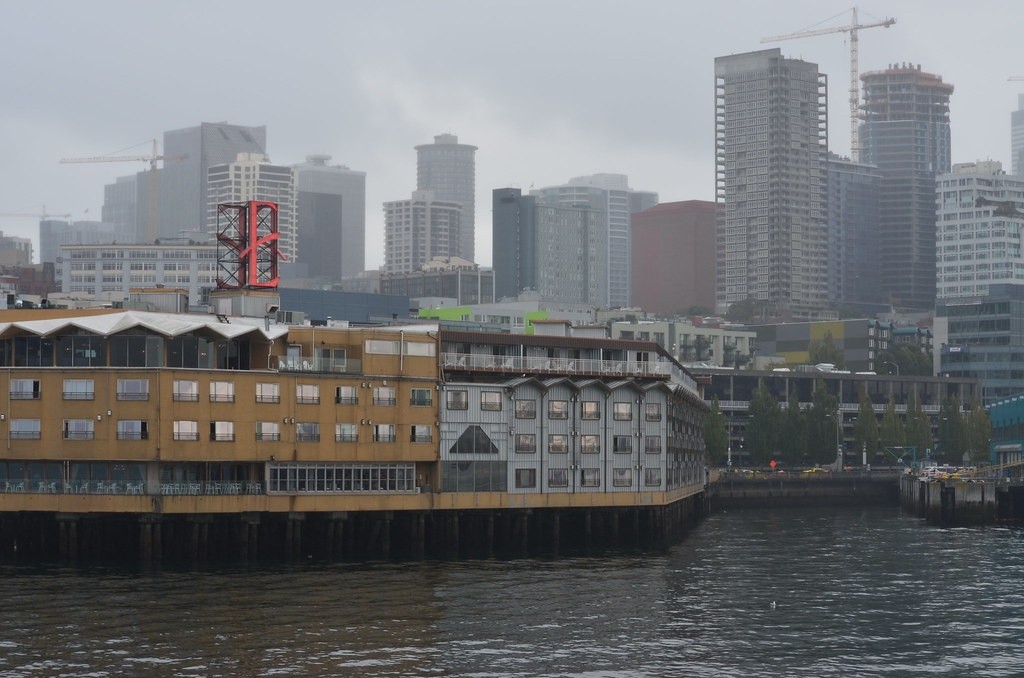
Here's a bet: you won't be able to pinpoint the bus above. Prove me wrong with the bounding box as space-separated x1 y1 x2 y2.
923 467 977 476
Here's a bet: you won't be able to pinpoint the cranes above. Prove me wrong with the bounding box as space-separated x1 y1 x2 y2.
58 138 190 245
759 6 897 163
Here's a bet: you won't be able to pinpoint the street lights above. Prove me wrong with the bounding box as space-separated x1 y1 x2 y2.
826 414 839 456
913 418 919 446
933 418 947 461
884 361 899 375
717 412 731 472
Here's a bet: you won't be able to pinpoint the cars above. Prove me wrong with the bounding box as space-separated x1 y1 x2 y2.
800 468 831 475
843 463 862 471
